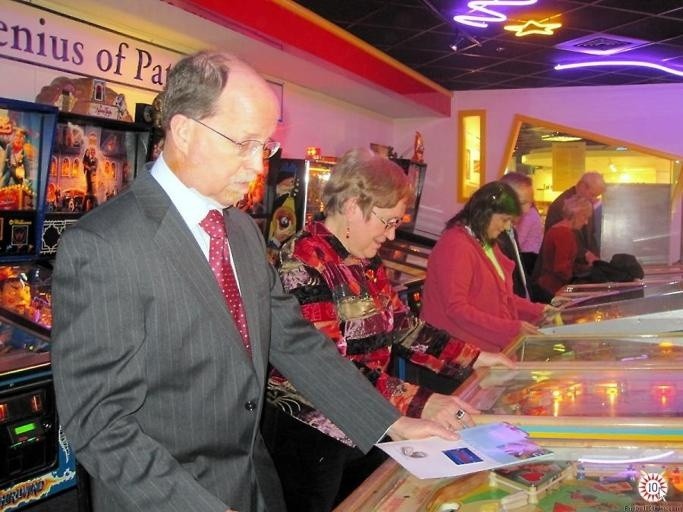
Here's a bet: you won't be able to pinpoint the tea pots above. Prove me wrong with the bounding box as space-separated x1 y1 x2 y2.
457 108 486 203
500 114 683 216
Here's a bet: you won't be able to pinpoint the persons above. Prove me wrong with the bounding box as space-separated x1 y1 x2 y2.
0 130 30 187
513 203 544 297
90 149 97 195
51 50 461 512
544 172 606 267
259 146 518 512
531 193 593 305
82 148 92 195
421 181 561 392
496 173 555 304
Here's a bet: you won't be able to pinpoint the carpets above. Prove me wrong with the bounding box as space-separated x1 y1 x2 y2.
199 209 251 361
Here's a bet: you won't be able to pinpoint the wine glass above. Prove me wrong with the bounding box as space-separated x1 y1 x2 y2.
456 408 466 420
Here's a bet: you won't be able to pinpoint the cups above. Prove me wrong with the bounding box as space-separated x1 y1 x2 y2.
182 112 280 159
487 182 506 201
369 210 403 230
